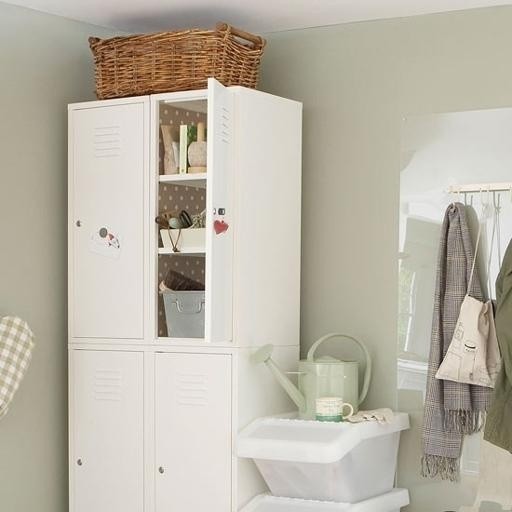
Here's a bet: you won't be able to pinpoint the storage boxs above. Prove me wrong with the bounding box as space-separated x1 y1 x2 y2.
235 412 410 512
158 290 205 338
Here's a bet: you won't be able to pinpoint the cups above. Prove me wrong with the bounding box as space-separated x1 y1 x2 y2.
315 396 354 422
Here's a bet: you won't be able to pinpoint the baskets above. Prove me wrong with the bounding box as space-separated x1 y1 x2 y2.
87 20 267 100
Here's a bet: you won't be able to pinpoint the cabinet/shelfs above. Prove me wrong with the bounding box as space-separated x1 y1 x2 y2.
67 78 305 512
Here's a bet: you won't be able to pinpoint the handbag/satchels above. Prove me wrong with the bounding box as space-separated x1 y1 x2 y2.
433 293 504 391
